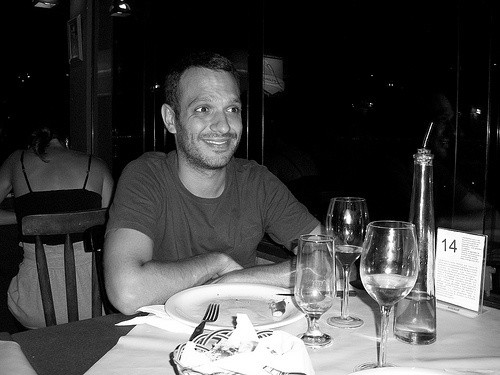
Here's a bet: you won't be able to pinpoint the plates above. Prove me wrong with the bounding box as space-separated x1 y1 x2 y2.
164 283 306 332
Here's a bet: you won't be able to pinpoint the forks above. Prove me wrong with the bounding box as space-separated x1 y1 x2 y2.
189 303 220 341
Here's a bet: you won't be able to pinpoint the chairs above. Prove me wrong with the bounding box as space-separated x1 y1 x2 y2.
22 208 109 326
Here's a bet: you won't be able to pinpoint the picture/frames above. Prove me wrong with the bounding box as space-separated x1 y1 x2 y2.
66 12 83 65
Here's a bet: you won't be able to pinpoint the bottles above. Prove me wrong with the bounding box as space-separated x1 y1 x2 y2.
393 149 438 346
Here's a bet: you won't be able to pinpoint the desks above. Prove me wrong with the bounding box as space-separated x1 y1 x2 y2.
0 281 500 375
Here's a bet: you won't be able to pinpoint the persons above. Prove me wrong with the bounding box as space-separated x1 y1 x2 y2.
0 112 113 329
271 130 321 214
427 95 500 230
104 54 354 316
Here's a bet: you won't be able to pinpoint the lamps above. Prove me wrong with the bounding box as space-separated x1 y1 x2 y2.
108 0 134 17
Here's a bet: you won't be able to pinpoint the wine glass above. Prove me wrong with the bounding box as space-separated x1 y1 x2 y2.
324 197 369 328
294 233 337 347
353 220 419 372
